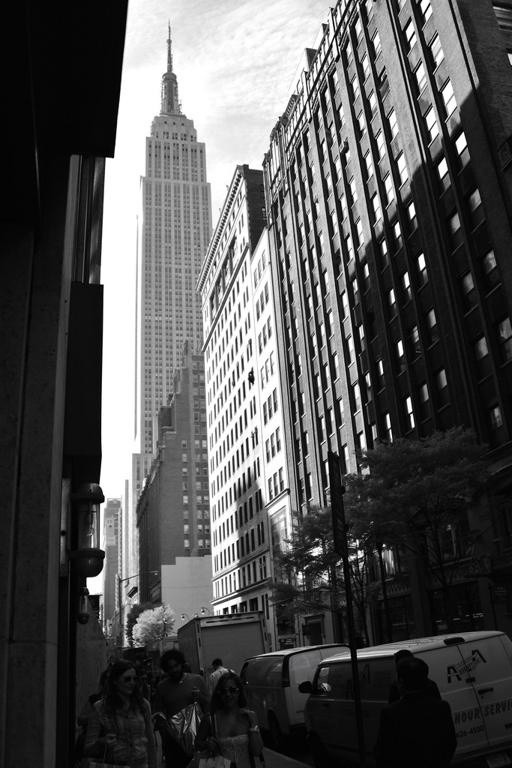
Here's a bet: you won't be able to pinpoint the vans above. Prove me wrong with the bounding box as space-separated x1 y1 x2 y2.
238 644 351 750
297 631 512 764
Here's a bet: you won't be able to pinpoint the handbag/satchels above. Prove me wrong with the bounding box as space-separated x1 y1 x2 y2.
198 752 232 768
164 702 206 755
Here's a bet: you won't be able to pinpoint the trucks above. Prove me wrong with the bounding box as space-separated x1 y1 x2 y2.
142 636 179 680
177 610 271 687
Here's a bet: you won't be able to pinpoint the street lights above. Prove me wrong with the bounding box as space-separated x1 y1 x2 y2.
117 571 159 646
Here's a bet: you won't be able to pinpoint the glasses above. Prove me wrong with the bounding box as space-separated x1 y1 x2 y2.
218 688 240 695
123 675 138 683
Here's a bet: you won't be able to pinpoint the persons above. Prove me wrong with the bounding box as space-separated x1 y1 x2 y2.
372 650 458 767
72 644 267 767
